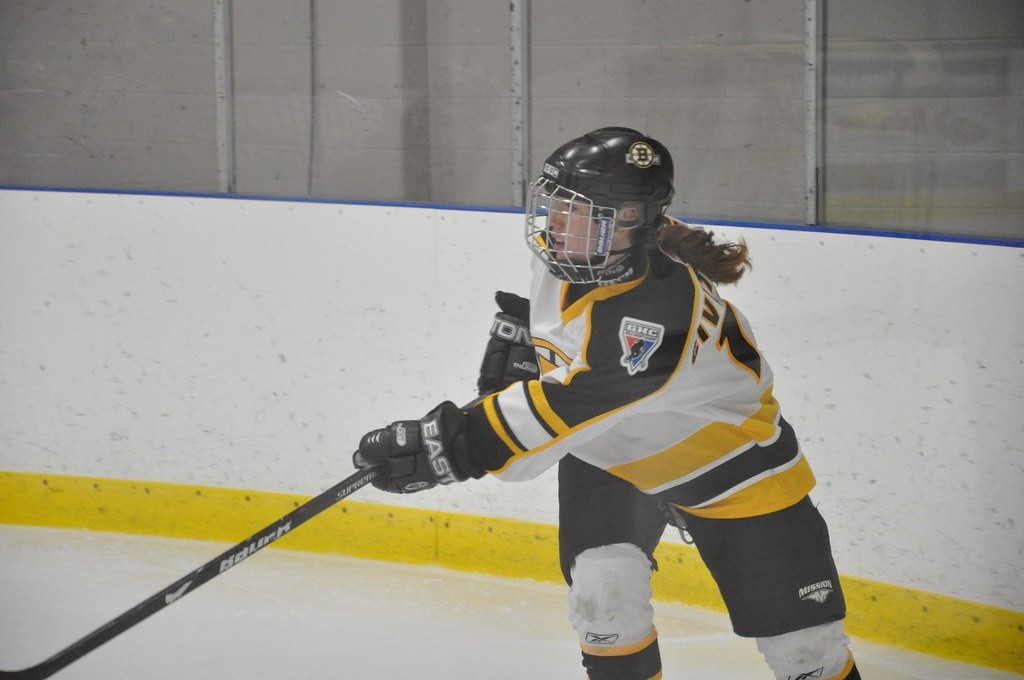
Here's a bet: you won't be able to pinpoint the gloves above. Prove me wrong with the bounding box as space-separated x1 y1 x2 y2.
477 291 540 399
353 401 487 495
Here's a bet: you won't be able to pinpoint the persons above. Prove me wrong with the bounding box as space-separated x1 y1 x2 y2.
352 126 861 680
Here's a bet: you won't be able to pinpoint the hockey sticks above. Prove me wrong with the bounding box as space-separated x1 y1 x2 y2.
0 386 499 680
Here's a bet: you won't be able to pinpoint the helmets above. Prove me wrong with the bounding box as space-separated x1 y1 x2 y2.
526 125 674 284
